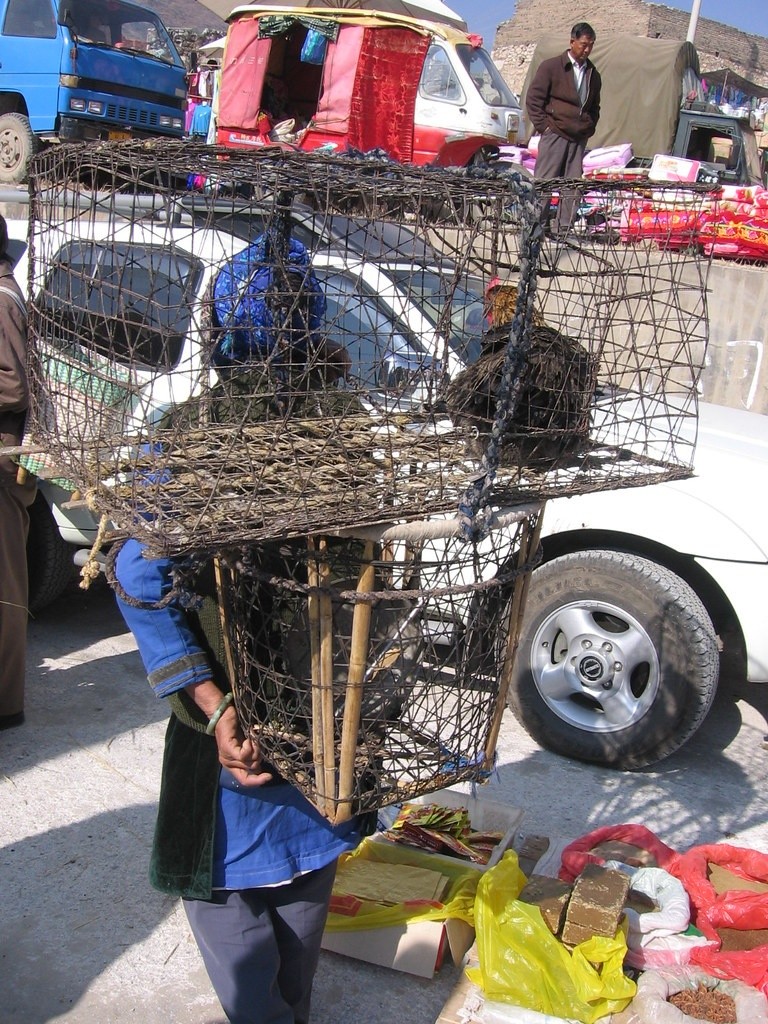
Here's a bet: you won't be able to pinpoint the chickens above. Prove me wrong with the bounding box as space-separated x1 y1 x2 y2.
445 278 611 451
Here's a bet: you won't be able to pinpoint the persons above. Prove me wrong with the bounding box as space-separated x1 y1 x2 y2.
525 22 602 243
82 10 107 45
105 233 405 1023
0 214 47 730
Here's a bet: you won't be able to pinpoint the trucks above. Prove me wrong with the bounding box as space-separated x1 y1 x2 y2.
0 1 198 185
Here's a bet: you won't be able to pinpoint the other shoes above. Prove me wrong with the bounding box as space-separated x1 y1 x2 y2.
0 712 25 731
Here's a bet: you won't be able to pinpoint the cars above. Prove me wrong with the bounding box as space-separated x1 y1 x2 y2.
0 195 767 773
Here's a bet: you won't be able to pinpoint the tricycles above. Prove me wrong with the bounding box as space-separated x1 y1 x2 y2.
214 6 540 236
518 30 764 191
